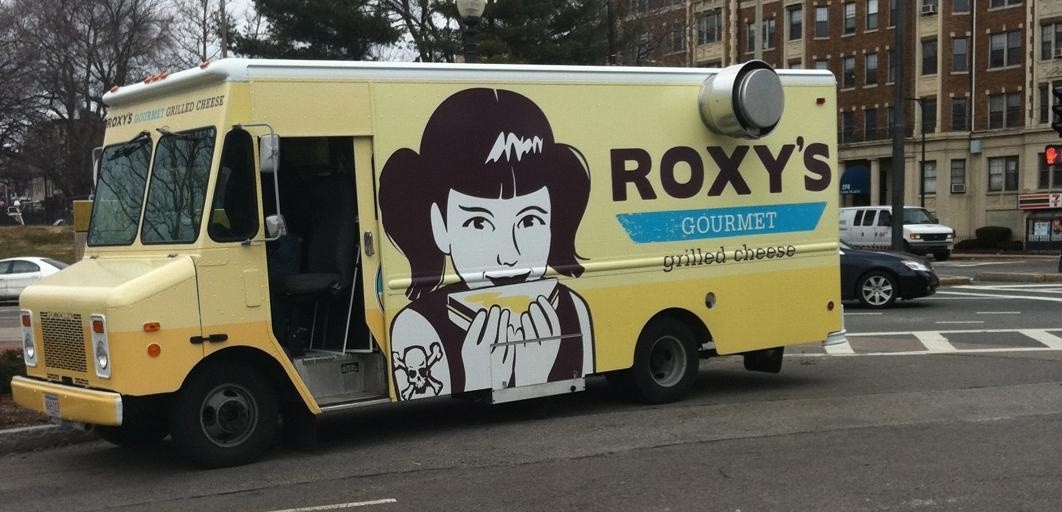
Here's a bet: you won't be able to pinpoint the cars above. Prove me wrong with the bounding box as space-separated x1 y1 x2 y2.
0 256 69 303
840 239 940 309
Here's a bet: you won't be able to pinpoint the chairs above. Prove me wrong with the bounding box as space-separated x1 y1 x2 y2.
269 175 359 349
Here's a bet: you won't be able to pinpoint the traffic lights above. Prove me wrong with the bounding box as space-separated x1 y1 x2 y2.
1045 145 1058 165
1051 87 1062 137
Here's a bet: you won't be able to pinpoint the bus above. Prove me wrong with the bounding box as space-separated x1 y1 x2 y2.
11 57 846 468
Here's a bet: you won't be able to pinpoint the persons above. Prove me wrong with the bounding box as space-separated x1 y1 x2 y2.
372 86 598 406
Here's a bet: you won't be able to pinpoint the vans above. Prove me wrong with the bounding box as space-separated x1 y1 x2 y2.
839 206 956 261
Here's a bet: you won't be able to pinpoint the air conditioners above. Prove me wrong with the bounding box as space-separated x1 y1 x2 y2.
920 4 937 14
951 184 965 193
